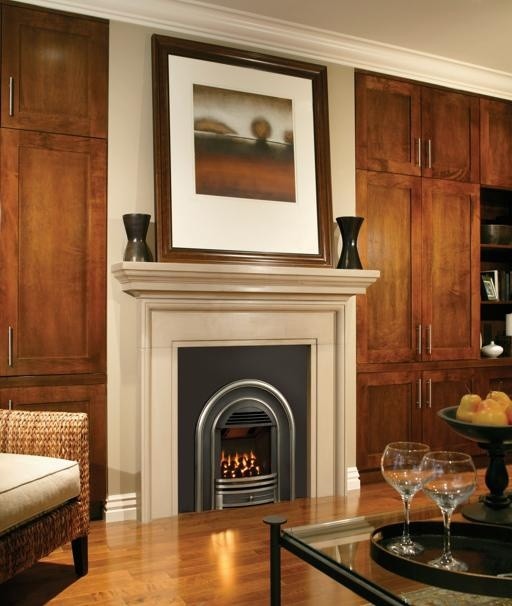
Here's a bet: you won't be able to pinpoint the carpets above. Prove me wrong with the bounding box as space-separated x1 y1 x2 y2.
364 586 512 606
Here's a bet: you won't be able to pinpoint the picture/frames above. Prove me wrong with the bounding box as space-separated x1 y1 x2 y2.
152 34 334 269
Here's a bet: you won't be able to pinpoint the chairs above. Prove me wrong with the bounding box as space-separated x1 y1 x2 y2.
0 410 90 585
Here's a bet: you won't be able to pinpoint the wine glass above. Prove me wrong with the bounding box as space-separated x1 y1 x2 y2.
436 406 512 525
380 441 430 560
420 450 479 573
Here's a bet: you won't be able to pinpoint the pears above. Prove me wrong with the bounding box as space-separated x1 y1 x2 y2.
457 383 512 425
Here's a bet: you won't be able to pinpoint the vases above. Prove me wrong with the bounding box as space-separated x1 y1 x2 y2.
123 214 153 262
336 217 364 269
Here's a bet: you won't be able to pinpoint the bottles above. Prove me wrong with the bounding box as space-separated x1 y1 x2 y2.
482 340 504 360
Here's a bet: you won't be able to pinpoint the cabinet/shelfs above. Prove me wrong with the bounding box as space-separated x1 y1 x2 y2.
1 0 109 520
355 71 512 484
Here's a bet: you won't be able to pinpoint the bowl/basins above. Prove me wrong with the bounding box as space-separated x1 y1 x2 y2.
480 224 512 245
